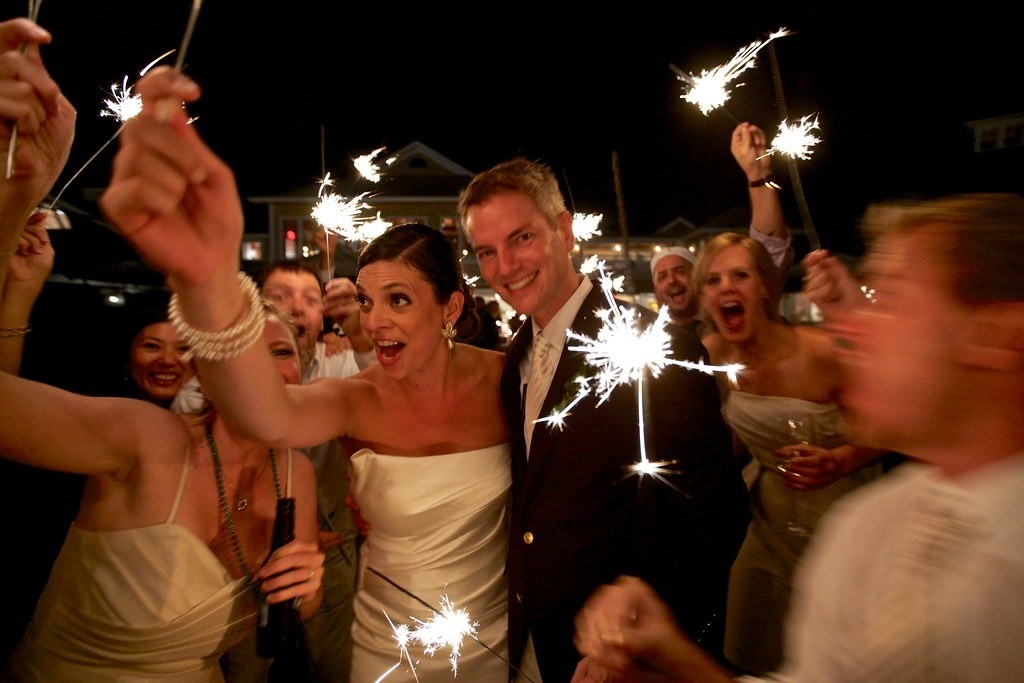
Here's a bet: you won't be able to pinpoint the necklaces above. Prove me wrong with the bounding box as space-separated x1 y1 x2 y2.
169 272 267 361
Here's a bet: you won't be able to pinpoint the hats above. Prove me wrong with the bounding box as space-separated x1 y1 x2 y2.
650 246 696 275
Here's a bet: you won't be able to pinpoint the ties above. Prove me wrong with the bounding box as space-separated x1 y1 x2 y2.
524 331 556 462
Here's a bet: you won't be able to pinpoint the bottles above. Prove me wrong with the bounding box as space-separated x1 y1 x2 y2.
256 494 298 659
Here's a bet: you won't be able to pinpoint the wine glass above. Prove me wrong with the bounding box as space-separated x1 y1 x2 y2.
774 411 816 537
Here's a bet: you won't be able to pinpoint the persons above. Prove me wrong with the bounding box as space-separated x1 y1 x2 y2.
651 120 910 682
105 65 541 682
459 159 754 683
0 209 378 683
580 189 1024 680
3 9 79 289
